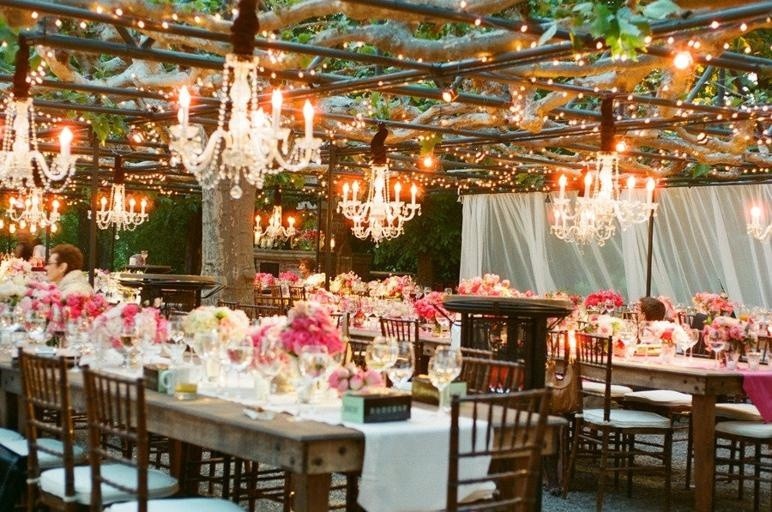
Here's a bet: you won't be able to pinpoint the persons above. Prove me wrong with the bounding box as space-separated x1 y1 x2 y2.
637 296 665 323
298 256 316 280
0 238 93 300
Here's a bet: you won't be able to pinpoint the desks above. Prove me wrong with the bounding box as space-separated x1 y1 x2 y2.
0 313 569 511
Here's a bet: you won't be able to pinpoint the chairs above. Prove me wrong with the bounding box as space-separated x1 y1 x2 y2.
15 345 183 510
80 363 248 510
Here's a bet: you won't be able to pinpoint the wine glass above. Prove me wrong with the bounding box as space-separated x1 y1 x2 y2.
574 300 725 369
0 306 462 421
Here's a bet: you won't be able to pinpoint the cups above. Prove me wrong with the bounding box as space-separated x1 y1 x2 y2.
746 352 761 370
724 353 741 369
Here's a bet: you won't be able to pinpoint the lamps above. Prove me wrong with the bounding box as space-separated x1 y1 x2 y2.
333 164 425 251
443 75 465 103
0 99 81 195
164 44 325 201
0 182 62 238
85 182 149 240
253 205 298 250
547 149 660 248
745 198 772 244
